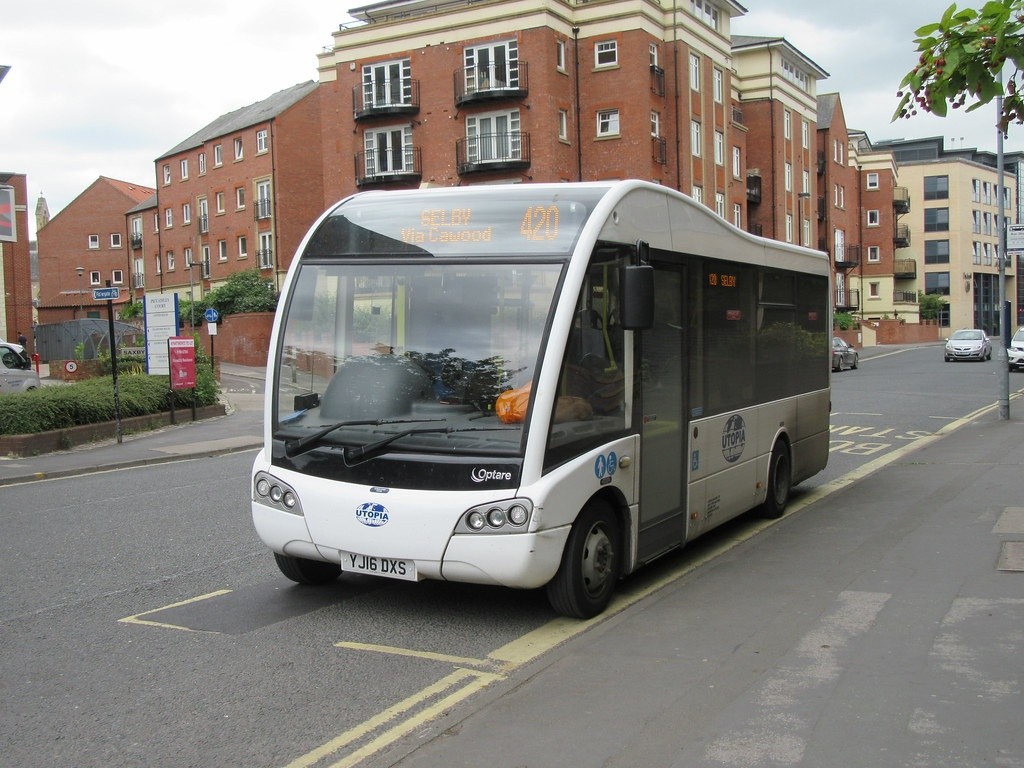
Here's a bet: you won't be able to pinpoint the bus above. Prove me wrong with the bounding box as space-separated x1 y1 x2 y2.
251 179 834 620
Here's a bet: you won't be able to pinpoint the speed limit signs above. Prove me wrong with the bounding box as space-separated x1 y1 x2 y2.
66 361 78 373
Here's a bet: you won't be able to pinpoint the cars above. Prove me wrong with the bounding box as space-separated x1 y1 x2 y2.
944 329 992 362
833 337 858 372
1007 327 1024 372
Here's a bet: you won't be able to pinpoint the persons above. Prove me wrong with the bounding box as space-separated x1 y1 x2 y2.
17 331 27 349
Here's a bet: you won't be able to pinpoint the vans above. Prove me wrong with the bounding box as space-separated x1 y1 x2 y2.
0 343 40 396
